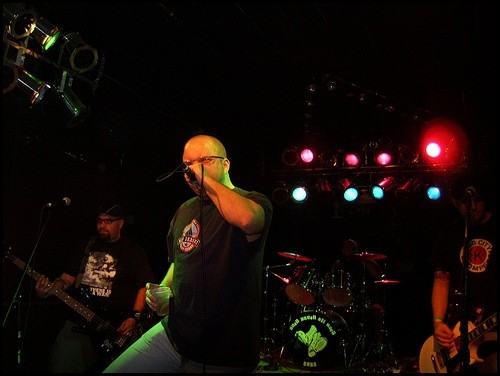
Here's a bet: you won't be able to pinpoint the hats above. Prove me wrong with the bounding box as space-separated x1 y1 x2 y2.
95 201 124 216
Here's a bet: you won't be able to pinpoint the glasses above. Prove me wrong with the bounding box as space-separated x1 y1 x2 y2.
183 155 225 166
96 217 123 225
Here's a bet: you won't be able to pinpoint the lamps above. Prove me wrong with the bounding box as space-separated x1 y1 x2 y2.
0 0 99 130
289 139 442 204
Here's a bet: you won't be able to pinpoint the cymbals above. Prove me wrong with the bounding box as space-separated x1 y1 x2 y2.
374 279 400 284
353 253 388 261
276 251 314 262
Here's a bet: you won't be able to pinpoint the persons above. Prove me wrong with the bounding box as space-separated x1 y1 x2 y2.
432 180 497 373
320 238 384 345
35 204 155 374
103 135 272 374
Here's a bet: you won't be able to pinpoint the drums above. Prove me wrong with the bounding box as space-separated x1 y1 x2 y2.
282 307 351 371
322 267 357 306
342 307 386 353
284 265 327 305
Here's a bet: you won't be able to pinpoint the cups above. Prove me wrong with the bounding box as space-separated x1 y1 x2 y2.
150 287 172 316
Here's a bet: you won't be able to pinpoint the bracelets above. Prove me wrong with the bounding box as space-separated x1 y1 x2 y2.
130 310 142 321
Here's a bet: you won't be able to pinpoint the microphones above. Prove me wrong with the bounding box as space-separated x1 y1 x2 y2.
181 163 204 193
48 197 71 207
464 186 477 200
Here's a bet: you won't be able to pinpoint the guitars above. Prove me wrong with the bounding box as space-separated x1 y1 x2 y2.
418 312 498 374
0 244 147 365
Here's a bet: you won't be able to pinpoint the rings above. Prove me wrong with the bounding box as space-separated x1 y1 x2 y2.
127 332 131 336
